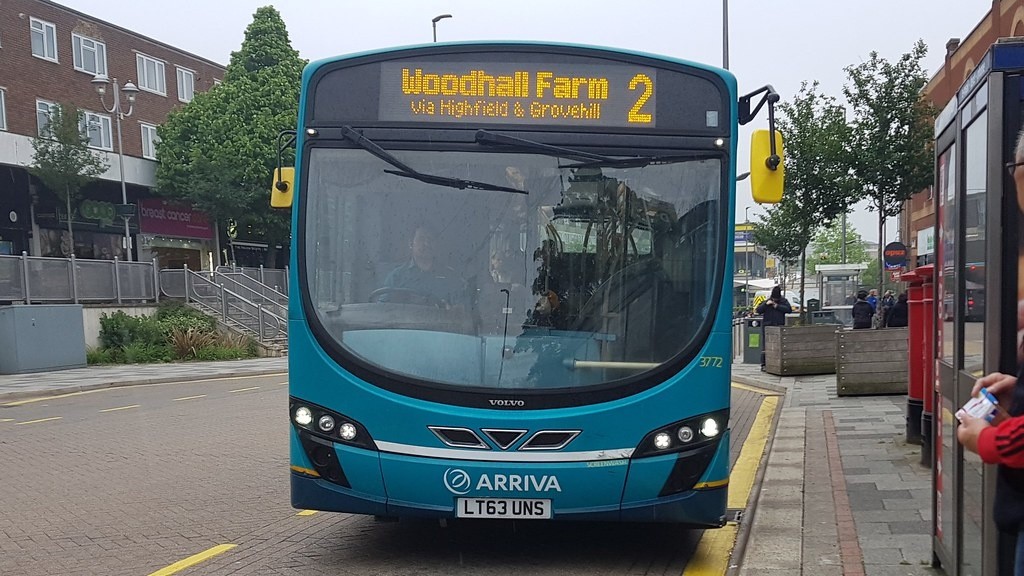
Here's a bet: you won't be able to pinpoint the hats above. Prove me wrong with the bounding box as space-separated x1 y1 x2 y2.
858 290 869 299
772 287 781 299
899 293 908 301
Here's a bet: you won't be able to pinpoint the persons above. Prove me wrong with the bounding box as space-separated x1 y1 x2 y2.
380 225 470 304
836 290 908 329
490 249 518 283
757 286 792 372
956 129 1024 576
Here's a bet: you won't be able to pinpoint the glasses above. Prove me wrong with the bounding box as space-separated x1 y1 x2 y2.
1006 161 1024 176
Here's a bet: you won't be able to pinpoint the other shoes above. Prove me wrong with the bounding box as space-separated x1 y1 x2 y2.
761 366 766 371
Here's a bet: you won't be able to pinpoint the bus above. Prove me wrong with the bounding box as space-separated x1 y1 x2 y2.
271 35 784 530
944 264 986 323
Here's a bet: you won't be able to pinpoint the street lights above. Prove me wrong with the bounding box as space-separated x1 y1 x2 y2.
91 71 140 261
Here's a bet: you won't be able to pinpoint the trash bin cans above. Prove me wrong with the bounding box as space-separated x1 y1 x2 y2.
813 311 838 326
744 317 764 363
807 298 821 322
